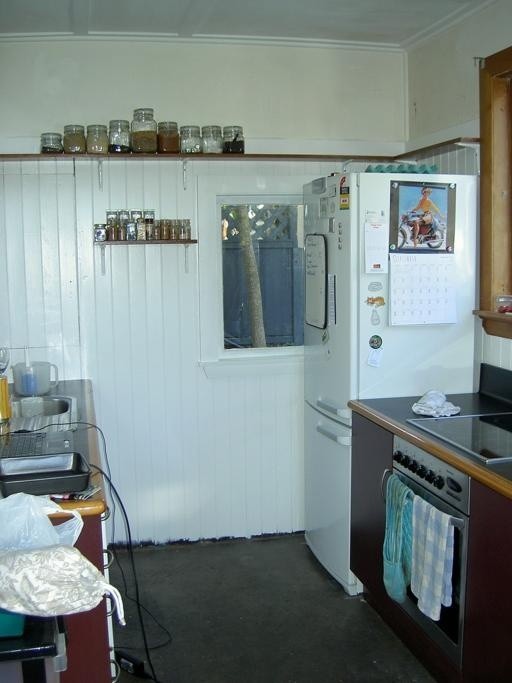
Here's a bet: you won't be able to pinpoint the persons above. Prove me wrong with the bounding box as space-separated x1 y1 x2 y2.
408 187 444 247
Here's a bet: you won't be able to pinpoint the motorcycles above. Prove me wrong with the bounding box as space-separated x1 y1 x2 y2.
398 210 444 248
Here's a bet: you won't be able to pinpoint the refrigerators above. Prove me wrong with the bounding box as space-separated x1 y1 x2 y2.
301 171 478 596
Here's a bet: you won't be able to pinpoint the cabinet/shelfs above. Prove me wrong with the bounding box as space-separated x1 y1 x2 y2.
0 152 399 244
0 377 122 683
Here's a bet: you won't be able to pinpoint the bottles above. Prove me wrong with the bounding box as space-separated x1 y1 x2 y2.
93 209 192 242
38 105 245 154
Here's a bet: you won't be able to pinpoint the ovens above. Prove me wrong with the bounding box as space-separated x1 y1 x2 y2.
385 433 472 673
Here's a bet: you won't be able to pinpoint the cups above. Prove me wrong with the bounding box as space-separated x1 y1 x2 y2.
10 360 60 397
20 396 44 416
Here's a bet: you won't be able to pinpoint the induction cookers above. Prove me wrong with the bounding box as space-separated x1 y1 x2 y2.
404 410 512 465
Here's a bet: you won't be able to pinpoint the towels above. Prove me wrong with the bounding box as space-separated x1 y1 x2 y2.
410 388 461 419
381 474 456 623
